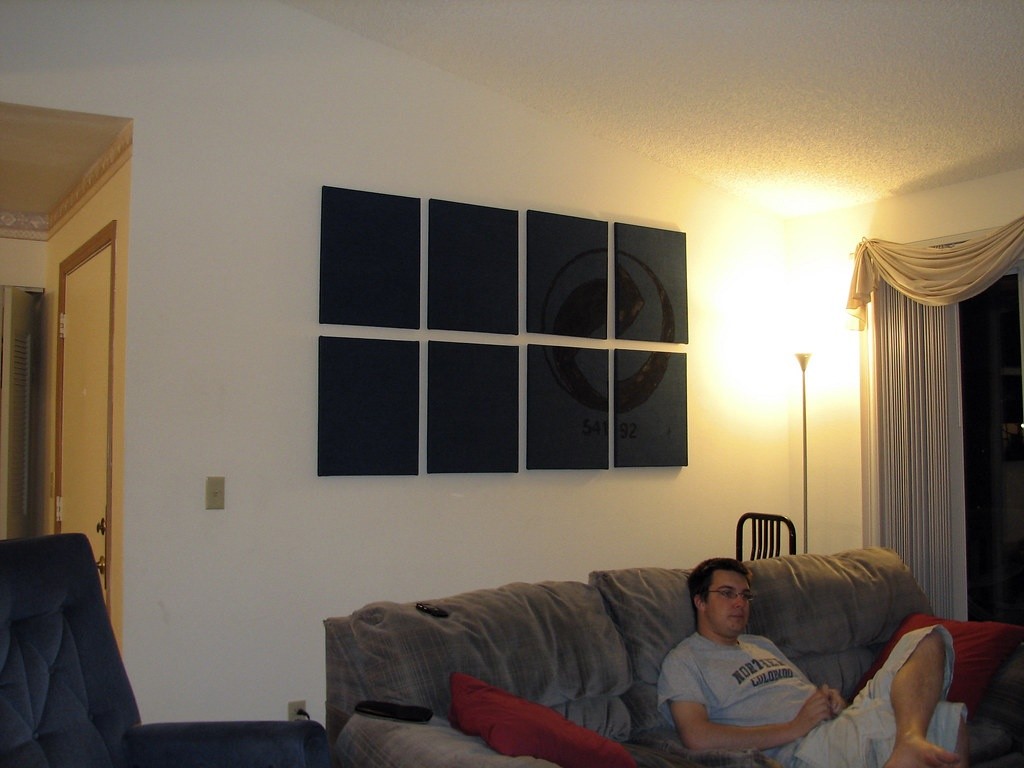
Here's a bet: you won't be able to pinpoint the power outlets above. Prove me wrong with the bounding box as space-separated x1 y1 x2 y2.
288 700 306 721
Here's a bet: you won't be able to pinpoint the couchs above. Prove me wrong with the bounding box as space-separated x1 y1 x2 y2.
322 545 1024 767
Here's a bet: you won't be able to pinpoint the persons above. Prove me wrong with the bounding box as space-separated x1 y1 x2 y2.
658 558 968 768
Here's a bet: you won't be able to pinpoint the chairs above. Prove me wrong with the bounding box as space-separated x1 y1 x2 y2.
0 533 331 768
734 513 797 560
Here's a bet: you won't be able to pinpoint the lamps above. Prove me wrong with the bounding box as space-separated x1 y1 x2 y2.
795 352 814 557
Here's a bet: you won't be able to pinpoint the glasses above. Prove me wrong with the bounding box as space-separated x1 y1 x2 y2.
709 589 754 601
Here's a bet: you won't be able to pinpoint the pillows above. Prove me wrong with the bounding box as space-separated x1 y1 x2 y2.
852 613 1024 712
444 670 637 768
